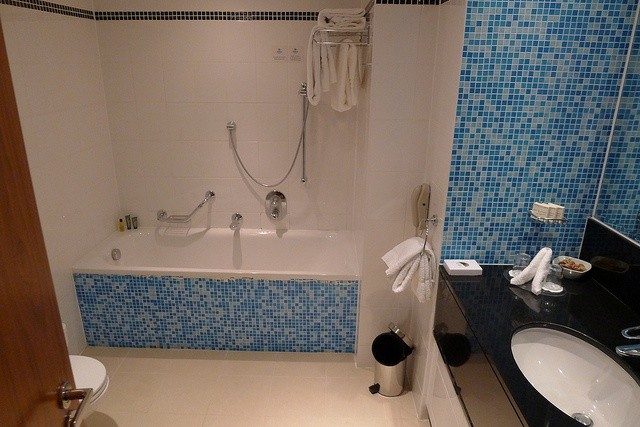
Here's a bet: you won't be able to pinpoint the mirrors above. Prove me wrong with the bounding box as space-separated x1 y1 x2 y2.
592 0 640 247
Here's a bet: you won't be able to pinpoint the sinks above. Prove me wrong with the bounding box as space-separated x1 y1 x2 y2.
511 322 639 427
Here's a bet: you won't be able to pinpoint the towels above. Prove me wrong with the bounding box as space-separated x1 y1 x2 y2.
316 8 367 30
306 26 335 106
508 246 552 295
391 253 431 303
380 236 437 287
332 38 358 112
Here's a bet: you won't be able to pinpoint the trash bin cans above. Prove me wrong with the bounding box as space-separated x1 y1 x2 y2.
369 320 415 396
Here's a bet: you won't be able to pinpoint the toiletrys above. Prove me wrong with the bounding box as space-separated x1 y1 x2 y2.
118 218 125 232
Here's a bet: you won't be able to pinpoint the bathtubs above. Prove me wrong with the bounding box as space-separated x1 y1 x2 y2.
73 226 359 354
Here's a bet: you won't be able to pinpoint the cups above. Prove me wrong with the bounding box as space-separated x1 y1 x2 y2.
514 253 530 278
543 265 562 292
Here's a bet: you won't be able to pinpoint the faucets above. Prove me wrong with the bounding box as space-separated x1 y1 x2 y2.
616 344 640 356
229 213 244 230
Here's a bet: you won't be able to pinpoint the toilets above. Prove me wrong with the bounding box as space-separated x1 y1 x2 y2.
62 323 110 419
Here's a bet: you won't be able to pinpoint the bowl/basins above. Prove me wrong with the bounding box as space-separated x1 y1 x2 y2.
553 255 591 277
